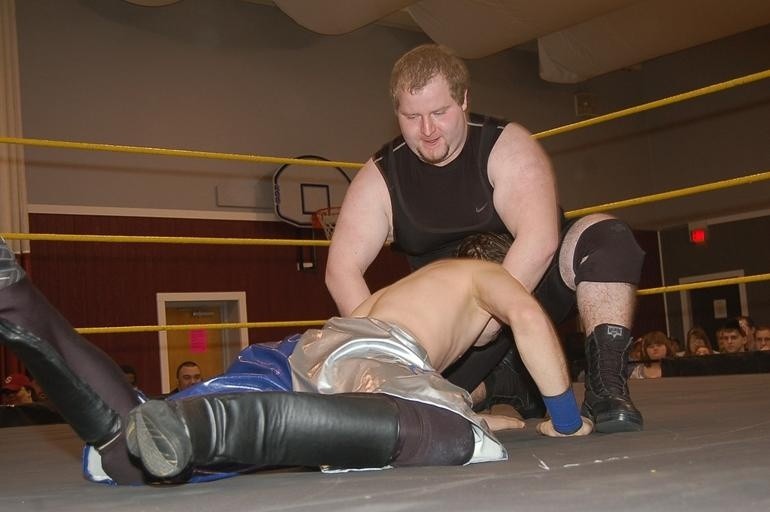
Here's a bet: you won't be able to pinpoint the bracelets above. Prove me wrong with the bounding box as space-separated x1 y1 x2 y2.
538 382 583 435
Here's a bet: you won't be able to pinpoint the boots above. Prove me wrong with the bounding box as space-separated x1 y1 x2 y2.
126 391 400 479
0 237 148 457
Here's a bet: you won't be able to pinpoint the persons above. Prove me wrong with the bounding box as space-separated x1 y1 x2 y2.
0 371 41 408
318 43 644 434
120 362 141 391
0 231 595 495
171 360 202 392
629 310 769 378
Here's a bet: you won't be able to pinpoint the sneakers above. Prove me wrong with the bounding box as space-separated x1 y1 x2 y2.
581 321 647 432
479 345 553 421
